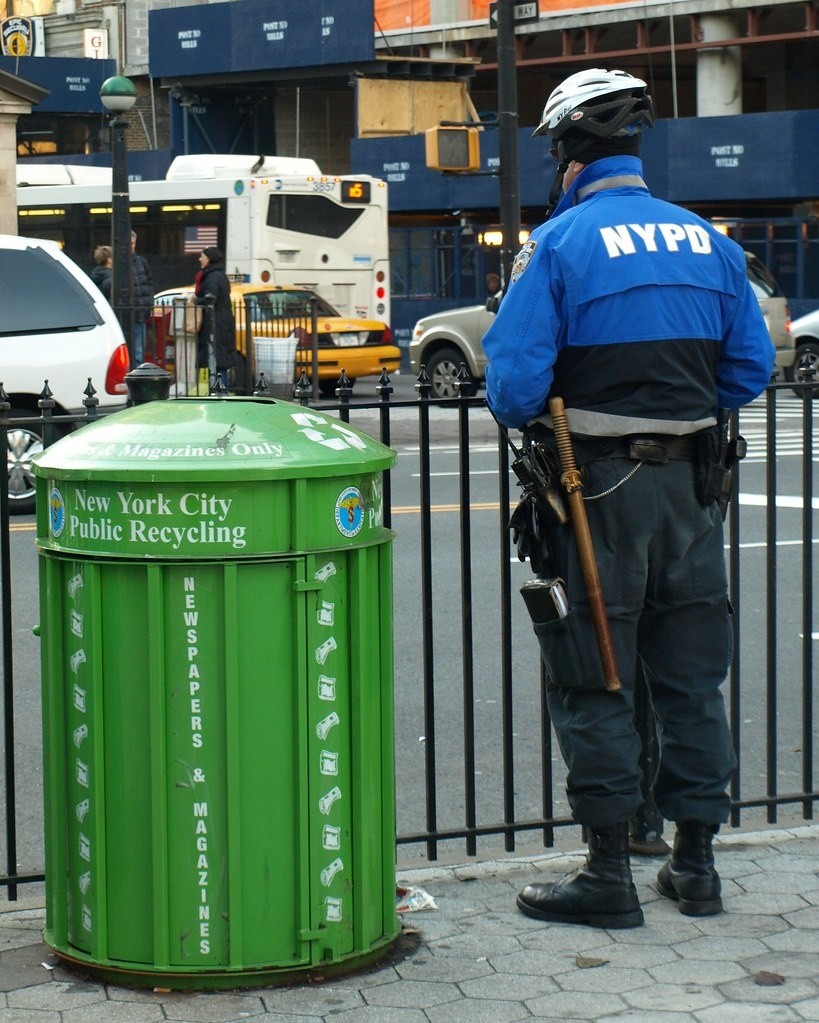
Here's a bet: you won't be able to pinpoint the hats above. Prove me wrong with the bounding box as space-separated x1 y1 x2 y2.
203 246 223 264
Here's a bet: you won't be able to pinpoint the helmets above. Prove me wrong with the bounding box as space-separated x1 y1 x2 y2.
531 68 655 139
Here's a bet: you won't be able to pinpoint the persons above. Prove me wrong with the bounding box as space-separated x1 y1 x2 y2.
88 230 153 370
188 247 237 397
481 68 777 926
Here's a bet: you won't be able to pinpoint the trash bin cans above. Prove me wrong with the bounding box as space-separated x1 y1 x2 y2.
30 396 401 989
252 337 299 401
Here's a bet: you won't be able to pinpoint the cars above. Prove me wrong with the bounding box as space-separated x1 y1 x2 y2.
783 310 819 400
150 280 401 397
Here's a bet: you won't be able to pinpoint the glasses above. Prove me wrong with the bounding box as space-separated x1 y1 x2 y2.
549 146 559 160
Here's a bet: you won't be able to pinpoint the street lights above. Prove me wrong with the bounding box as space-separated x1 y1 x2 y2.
98 77 137 372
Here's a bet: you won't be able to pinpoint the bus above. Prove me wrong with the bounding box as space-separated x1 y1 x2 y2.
16 154 390 326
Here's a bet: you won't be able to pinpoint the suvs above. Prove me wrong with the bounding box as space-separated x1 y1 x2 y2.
409 250 795 408
0 233 131 514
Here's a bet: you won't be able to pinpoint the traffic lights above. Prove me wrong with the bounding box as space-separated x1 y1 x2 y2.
424 125 476 174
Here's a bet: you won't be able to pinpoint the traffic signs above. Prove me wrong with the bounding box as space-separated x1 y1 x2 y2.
488 0 539 31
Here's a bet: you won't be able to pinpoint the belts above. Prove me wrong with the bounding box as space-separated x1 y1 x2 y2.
577 433 617 458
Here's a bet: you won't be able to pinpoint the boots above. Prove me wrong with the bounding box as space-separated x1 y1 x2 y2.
516 823 645 929
657 822 723 917
